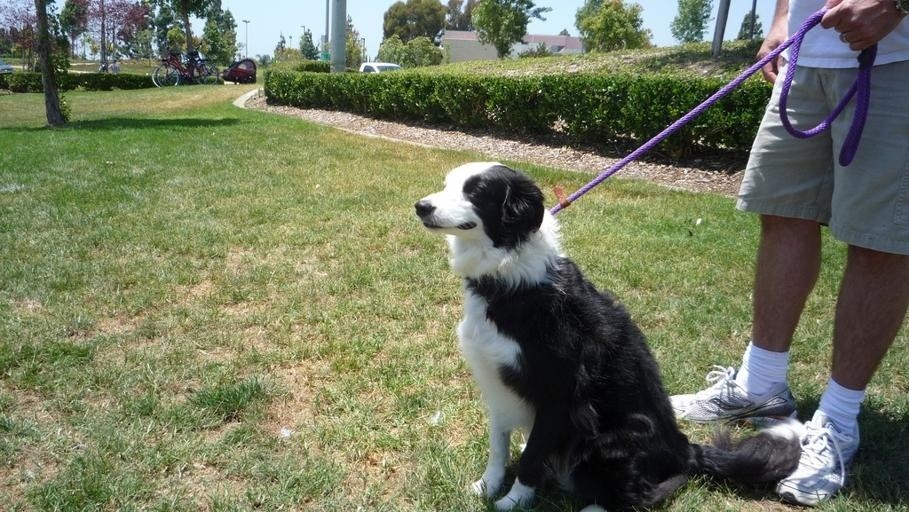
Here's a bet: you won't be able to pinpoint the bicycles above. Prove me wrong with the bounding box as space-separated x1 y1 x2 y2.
151 46 219 88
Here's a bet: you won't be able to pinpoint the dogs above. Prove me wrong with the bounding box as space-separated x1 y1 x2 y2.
414 160 808 512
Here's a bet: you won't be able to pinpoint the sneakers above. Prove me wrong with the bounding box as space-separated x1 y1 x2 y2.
667 364 797 425
775 410 860 508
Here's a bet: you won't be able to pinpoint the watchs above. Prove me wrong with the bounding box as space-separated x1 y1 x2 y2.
895 0 909 14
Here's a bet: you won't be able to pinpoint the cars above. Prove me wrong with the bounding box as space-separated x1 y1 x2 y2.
223 59 256 83
0 59 15 72
359 62 401 73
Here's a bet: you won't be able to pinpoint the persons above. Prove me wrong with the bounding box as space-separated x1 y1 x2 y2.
99 61 108 72
666 0 909 508
108 60 121 74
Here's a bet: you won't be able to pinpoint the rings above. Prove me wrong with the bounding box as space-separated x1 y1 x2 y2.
842 32 851 43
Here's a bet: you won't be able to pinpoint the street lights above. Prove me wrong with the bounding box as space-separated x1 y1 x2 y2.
243 20 251 58
